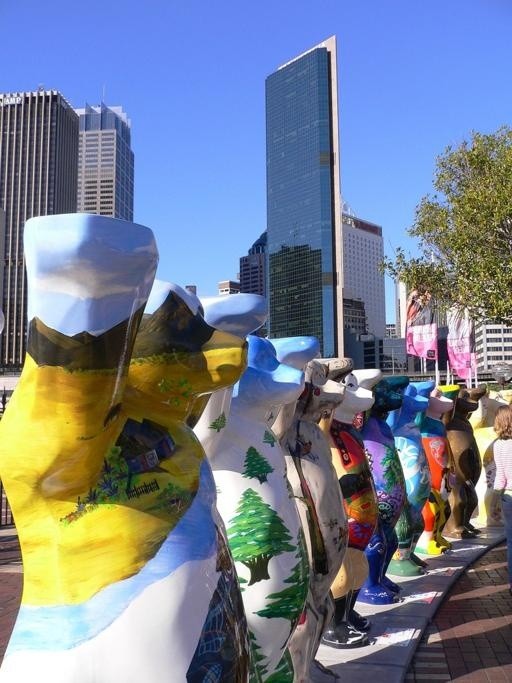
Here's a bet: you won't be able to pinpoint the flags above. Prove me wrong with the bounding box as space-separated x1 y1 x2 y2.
442 306 478 379
403 283 438 360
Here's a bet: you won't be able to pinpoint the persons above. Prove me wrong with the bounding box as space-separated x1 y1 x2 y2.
491 403 512 588
